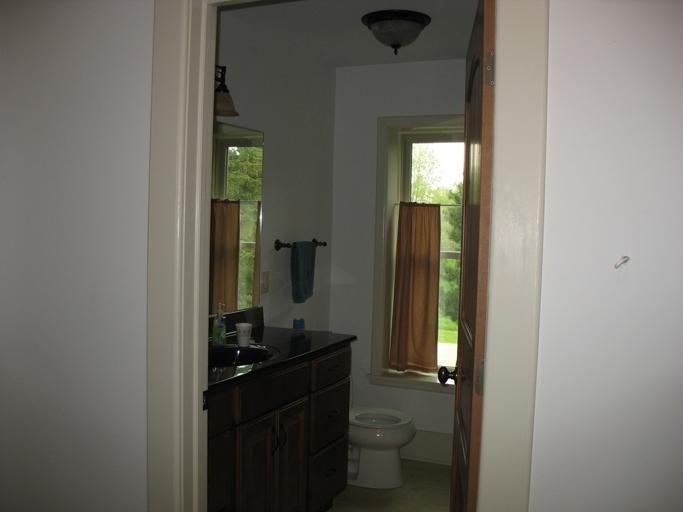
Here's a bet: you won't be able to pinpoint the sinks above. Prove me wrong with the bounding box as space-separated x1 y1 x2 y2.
208 344 270 369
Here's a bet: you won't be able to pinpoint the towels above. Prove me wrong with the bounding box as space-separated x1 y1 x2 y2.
291 241 316 303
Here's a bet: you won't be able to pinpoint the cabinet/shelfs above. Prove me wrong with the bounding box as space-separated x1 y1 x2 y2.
209 346 350 511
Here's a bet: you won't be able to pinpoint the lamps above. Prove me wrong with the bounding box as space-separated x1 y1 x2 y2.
214 64 239 118
360 10 431 57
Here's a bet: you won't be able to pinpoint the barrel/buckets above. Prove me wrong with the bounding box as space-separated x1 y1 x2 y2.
347 375 418 488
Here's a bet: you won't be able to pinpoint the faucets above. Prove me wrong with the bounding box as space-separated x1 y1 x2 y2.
232 349 242 376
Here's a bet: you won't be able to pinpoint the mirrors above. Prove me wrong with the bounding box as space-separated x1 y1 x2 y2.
208 122 264 317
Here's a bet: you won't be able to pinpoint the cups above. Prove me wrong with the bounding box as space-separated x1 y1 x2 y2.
236 323 252 347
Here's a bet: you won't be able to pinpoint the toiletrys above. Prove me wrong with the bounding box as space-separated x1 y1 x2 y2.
212 302 228 345
